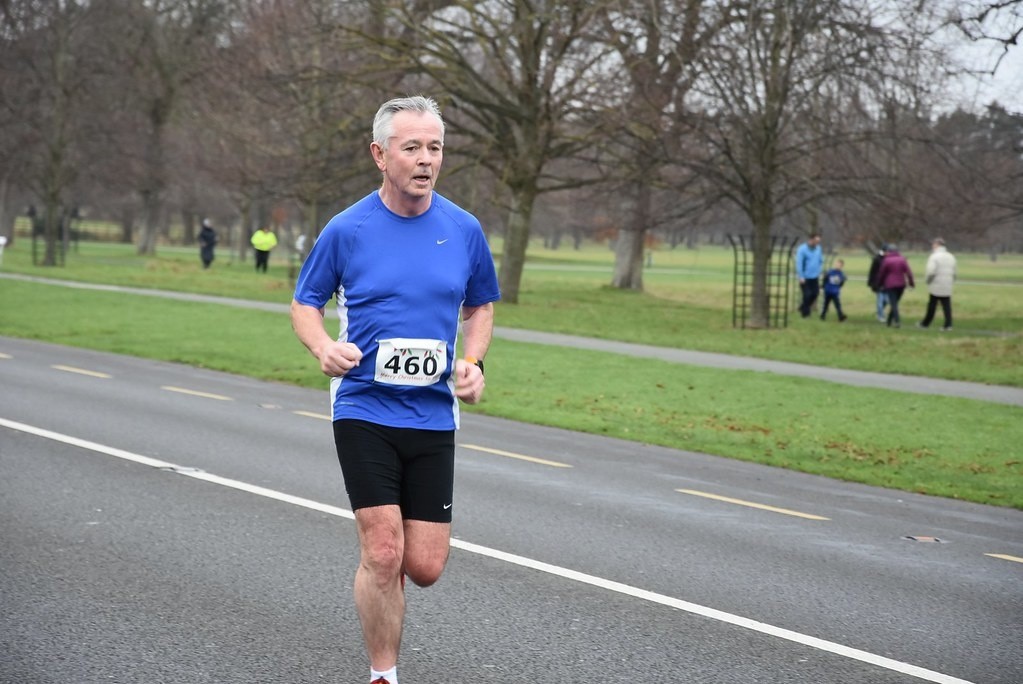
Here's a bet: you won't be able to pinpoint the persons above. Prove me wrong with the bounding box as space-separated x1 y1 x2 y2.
820 259 847 322
795 232 824 318
868 243 915 328
290 95 500 684
199 219 217 269
916 237 957 331
251 225 277 273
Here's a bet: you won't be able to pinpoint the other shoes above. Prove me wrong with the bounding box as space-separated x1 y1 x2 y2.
894 322 901 329
915 321 927 331
839 315 846 320
820 315 827 322
940 326 953 331
878 316 886 322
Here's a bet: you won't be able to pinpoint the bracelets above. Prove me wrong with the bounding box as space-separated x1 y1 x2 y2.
464 358 483 374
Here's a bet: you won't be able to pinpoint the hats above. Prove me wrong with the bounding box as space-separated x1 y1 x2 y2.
929 236 946 246
881 243 889 251
